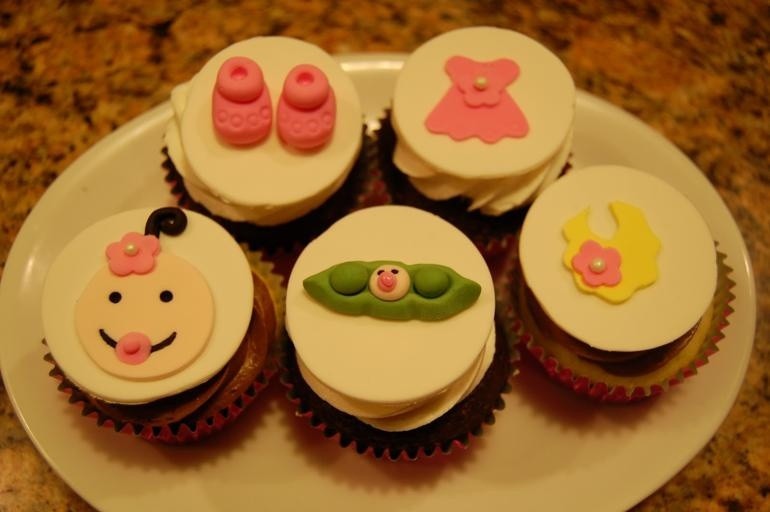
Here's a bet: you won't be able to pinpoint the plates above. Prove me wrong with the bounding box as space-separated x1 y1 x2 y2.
0 52 757 511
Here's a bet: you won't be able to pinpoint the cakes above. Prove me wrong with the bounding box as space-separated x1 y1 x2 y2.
42 205 278 429
161 36 374 227
391 24 576 217
515 163 722 379
286 205 497 435
